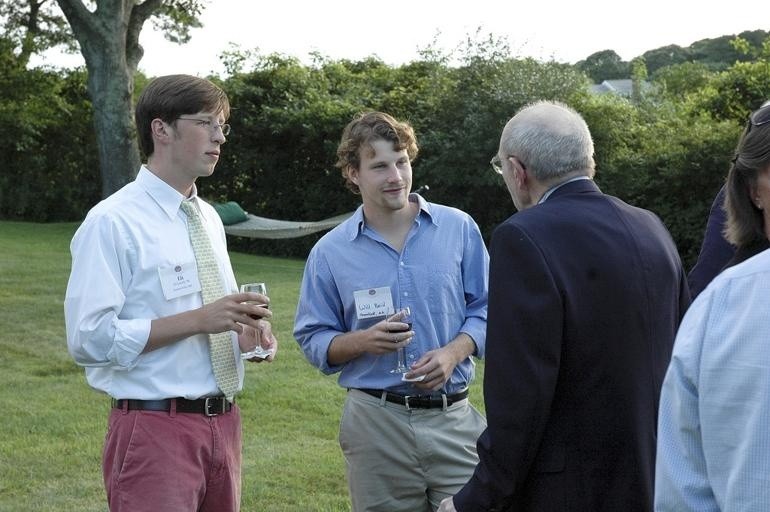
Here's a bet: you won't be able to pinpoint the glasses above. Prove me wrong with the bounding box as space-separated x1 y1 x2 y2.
177 118 232 136
745 104 770 136
490 155 526 175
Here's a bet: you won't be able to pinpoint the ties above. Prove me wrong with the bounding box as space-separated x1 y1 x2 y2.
180 199 241 404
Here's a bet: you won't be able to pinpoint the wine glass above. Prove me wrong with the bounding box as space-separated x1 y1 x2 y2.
385 307 412 373
240 281 274 358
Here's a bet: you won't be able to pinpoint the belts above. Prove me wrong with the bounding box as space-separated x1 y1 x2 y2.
359 388 470 409
112 396 233 418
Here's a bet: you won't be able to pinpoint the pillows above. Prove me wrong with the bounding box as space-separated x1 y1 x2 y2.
211 201 248 225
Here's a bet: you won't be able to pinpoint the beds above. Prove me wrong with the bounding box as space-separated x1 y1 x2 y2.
207 200 360 239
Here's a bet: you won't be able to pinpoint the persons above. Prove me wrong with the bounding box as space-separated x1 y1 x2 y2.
291 111 491 512
63 72 278 511
716 98 770 272
685 180 736 301
652 247 770 512
436 100 693 512
